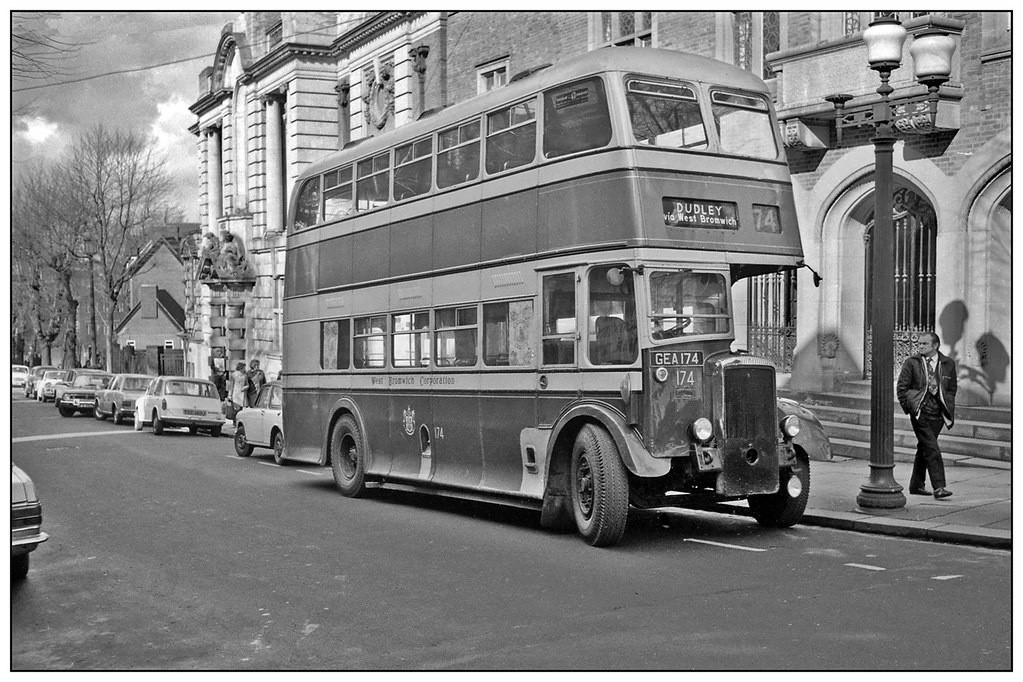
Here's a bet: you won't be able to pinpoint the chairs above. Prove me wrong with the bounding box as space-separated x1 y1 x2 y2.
595 316 632 366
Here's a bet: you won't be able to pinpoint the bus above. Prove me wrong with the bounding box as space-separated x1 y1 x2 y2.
280 44 834 550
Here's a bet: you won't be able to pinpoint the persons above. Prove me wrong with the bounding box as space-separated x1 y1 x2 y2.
226 362 250 427
897 332 958 499
246 360 266 408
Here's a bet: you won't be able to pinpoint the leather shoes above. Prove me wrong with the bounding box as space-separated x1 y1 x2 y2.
933 486 953 499
910 488 933 496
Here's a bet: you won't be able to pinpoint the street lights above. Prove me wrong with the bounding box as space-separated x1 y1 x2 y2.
822 10 961 519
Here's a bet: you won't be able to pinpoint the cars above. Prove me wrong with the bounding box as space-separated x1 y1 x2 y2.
36 370 61 402
24 365 57 399
12 461 51 580
133 376 228 437
56 368 94 403
12 363 29 386
57 371 115 420
90 372 157 426
232 380 287 465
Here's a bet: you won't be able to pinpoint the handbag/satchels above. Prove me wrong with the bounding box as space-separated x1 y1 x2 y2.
221 398 237 420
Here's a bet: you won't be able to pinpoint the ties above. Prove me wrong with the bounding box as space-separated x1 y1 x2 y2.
927 357 933 376
